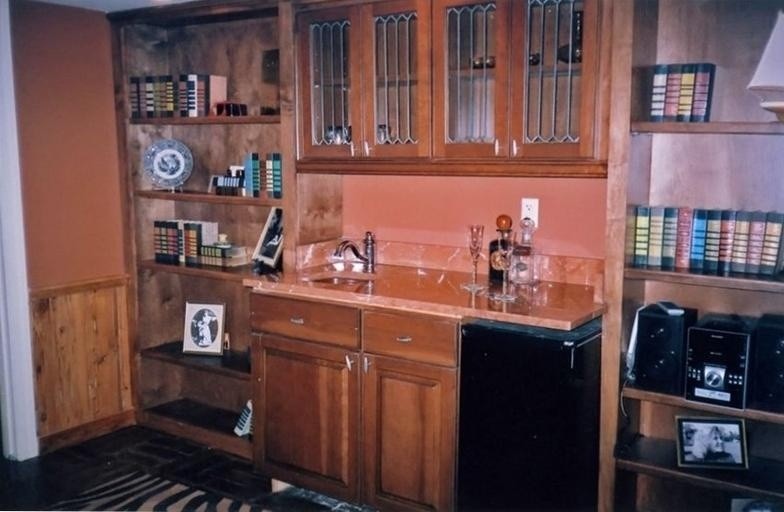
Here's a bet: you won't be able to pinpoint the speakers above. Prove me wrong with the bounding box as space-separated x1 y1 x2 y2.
746 312 784 416
636 302 695 396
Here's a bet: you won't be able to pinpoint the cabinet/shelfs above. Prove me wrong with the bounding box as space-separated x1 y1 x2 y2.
292 0 432 176
431 0 609 177
110 0 343 461
247 282 460 512
597 1 784 512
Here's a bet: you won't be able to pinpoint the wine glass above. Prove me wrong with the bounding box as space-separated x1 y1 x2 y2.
492 231 519 304
464 222 484 293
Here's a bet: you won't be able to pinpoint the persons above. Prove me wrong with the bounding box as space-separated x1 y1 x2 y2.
688 425 735 464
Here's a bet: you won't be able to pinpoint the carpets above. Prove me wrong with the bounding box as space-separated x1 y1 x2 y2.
30 467 275 512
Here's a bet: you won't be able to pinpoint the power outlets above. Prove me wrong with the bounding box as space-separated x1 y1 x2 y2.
521 198 539 228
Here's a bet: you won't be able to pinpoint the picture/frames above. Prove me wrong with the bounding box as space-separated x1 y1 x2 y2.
182 300 226 356
675 414 749 470
250 205 284 269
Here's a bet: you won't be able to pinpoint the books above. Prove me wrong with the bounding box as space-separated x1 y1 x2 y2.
129 73 227 118
642 63 715 122
633 205 784 276
234 399 253 437
151 220 249 267
246 152 283 198
